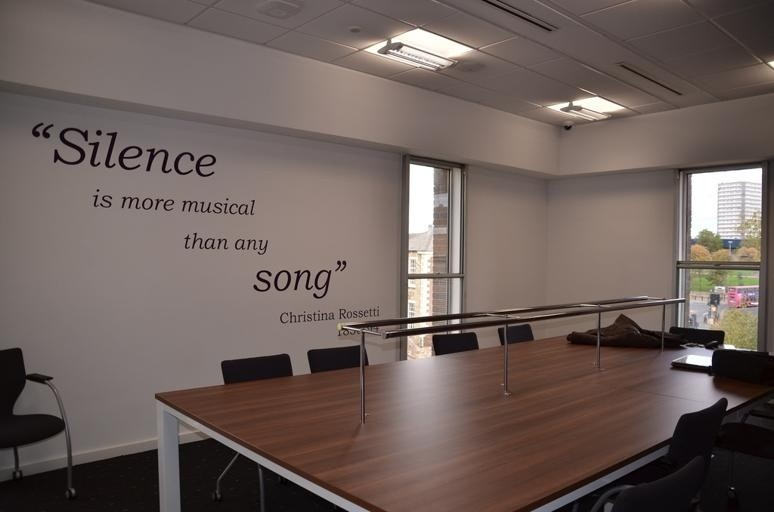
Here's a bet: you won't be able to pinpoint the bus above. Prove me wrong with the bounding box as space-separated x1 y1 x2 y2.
725 284 759 309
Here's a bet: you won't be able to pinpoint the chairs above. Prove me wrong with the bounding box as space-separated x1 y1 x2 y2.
0 347 77 498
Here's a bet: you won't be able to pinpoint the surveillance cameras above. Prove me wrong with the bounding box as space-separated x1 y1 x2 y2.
562 121 575 130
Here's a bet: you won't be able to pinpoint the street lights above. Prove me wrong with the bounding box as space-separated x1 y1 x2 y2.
727 241 733 257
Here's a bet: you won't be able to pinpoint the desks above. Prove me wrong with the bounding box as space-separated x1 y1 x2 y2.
154 334 774 512
